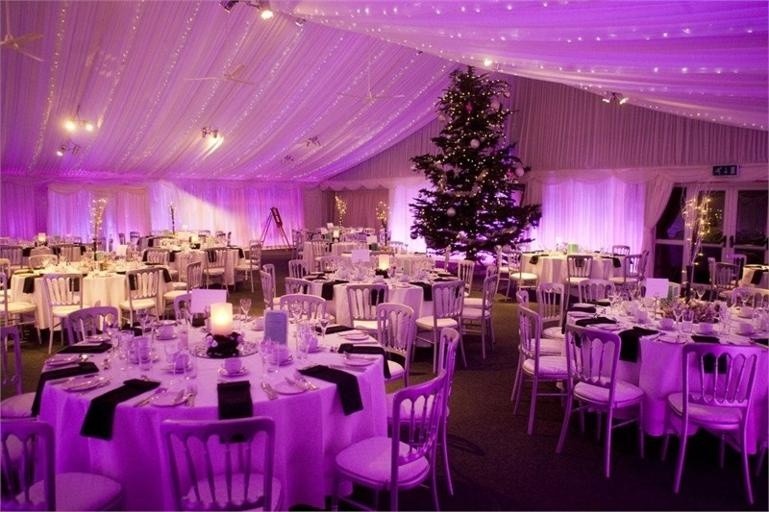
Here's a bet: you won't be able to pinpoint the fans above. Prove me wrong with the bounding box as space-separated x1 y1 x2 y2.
335 38 405 105
186 16 255 85
0 0 46 62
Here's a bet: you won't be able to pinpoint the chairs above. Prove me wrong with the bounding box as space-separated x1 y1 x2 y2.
502 229 769 314
331 369 450 512
510 289 565 407
1 325 36 417
375 302 416 388
1 418 124 512
554 322 645 478
512 304 584 436
160 416 283 512
386 327 461 496
1 229 501 372
659 342 763 505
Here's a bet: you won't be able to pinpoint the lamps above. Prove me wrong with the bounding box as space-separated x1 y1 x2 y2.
219 1 273 20
602 92 627 105
201 126 218 138
56 146 65 157
66 106 89 131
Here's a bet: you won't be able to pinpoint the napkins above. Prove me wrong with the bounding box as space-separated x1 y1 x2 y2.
298 364 365 417
79 378 161 442
216 380 254 444
691 335 727 374
753 338 768 347
31 362 99 418
618 326 659 363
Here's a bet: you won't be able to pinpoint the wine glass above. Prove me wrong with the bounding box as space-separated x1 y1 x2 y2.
104 298 328 385
606 285 768 335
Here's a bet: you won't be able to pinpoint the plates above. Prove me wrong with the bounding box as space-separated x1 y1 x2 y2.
567 288 769 346
47 313 375 408
311 269 456 287
